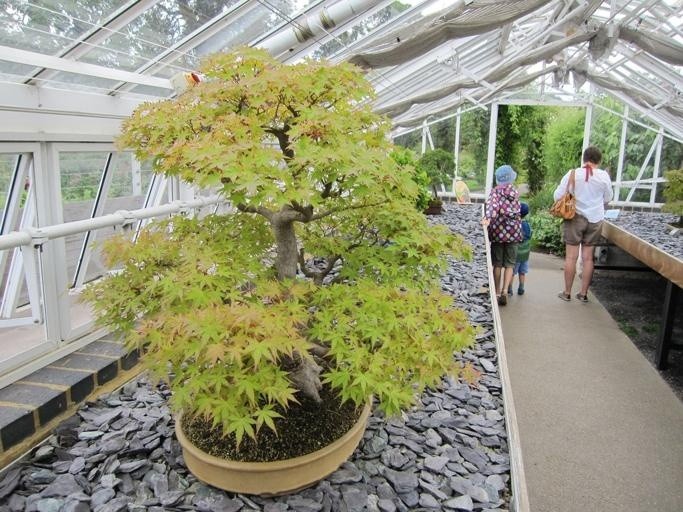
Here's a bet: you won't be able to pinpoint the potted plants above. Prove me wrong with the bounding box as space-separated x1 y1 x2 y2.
74 45 487 497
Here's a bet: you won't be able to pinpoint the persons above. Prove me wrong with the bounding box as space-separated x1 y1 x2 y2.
552 146 614 303
507 201 533 296
483 164 522 305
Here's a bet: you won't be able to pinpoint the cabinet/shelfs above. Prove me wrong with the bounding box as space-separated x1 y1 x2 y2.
601 216 683 370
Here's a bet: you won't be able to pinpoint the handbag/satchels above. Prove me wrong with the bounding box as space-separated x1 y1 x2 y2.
550 192 576 220
517 243 529 261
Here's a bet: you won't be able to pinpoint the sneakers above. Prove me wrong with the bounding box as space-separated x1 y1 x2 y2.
496 288 524 305
575 292 588 302
559 291 570 301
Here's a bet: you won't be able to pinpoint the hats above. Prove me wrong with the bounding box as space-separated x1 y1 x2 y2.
495 165 516 185
